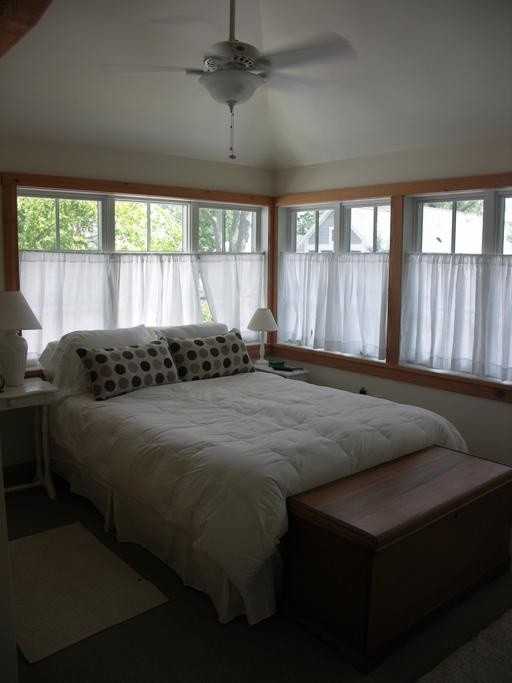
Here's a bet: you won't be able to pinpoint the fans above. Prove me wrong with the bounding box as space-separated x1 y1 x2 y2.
98 1 350 100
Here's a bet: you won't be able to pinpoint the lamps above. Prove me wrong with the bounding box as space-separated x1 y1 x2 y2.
191 67 267 161
247 307 280 366
0 290 44 386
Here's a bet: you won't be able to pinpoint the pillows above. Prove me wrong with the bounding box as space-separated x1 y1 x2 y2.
44 319 255 402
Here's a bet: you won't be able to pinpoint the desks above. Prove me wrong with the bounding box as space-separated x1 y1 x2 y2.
0 375 57 505
283 445 511 681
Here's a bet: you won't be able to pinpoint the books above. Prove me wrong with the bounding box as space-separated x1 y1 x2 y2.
273 365 305 372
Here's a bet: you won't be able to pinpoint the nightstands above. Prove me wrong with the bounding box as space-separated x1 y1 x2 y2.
255 362 311 384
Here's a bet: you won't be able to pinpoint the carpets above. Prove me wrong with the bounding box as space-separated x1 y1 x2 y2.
8 521 170 665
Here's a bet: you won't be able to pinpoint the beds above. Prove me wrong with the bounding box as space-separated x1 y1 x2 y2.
43 373 453 624
